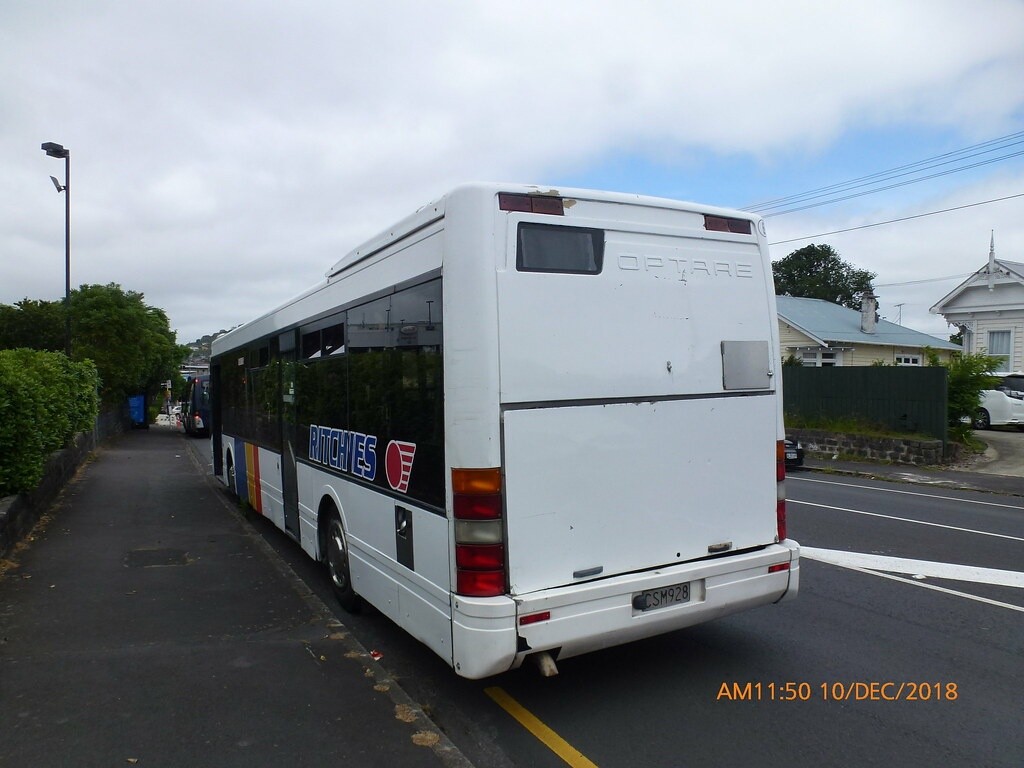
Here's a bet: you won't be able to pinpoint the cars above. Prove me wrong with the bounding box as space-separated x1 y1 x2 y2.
171 404 181 419
952 367 1024 430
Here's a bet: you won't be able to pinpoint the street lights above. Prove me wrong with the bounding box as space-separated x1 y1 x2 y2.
42 140 74 365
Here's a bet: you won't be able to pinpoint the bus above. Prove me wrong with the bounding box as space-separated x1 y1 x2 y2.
181 368 212 439
199 174 803 691
